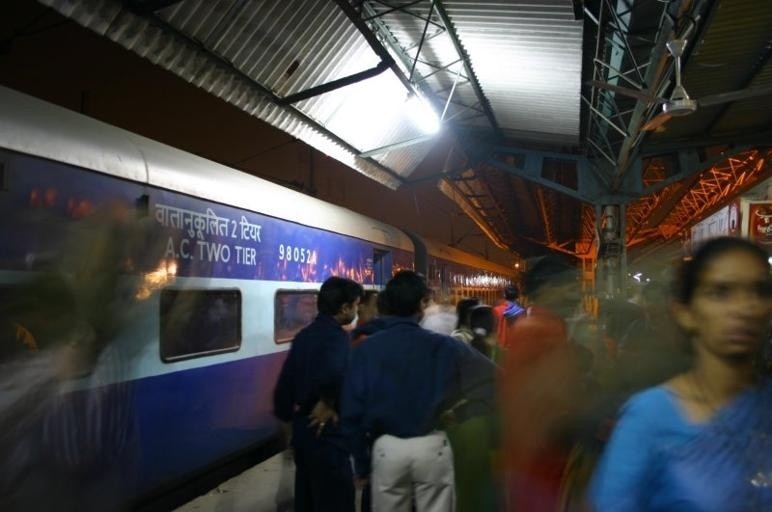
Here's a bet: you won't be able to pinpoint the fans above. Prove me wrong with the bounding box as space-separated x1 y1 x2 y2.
583 39 772 133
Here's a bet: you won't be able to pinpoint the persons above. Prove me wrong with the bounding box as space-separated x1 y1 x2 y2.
271 235 772 511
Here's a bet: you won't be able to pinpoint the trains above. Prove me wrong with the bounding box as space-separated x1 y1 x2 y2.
1 77 519 512
